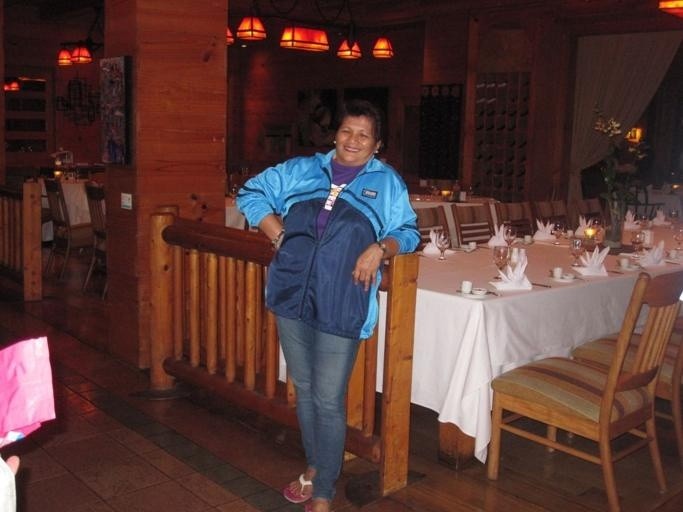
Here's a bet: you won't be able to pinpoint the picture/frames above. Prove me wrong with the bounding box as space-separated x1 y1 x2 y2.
99 55 131 166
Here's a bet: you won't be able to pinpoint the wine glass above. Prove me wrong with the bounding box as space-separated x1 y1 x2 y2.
551 218 564 245
226 174 240 204
570 238 586 266
631 232 644 258
493 246 510 279
672 227 682 249
503 226 517 246
436 231 450 260
640 216 647 226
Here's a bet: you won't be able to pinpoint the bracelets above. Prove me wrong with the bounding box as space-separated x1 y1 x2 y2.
271 229 285 250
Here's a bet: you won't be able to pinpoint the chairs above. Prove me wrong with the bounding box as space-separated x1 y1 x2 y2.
81 183 108 300
43 174 94 281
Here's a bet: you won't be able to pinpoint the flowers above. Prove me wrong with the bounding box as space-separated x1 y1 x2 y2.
592 102 650 238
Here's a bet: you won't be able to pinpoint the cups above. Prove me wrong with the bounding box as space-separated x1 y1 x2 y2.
524 235 532 243
620 258 629 268
553 266 562 279
459 192 467 201
469 242 477 249
461 281 472 293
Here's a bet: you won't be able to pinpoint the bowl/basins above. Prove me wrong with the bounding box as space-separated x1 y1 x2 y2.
472 288 487 295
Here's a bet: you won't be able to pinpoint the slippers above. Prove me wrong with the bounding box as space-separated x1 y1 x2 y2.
305 500 313 512
283 474 313 504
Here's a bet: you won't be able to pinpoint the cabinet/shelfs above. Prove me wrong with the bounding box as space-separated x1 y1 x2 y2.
471 3 568 204
418 81 464 180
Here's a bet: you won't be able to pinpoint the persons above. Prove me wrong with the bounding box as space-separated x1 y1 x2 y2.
235 99 421 512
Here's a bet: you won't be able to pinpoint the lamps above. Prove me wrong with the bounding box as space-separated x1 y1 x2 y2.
56 48 73 67
237 15 267 42
70 46 93 65
279 27 330 55
227 27 234 45
335 40 363 62
371 38 393 60
657 0 683 17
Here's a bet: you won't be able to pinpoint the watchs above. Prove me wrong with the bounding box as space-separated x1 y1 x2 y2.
374 242 387 257
